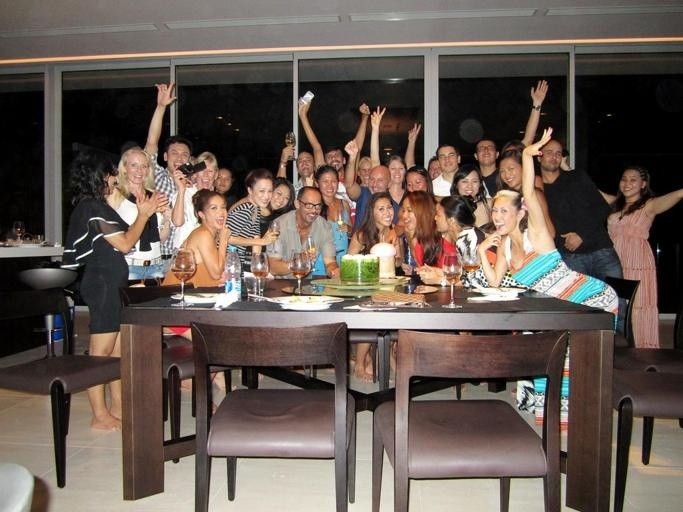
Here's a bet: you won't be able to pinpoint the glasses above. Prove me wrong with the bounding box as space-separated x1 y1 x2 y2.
301 201 322 210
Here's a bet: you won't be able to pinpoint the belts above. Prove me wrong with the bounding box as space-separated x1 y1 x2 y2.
127 257 163 266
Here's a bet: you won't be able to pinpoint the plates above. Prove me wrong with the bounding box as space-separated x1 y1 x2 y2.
167 291 232 305
412 284 438 295
471 286 527 295
266 296 345 307
309 277 408 289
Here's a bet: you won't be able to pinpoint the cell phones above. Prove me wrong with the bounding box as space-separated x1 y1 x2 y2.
301 91 315 106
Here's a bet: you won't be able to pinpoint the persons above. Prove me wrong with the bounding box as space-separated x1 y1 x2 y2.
590 166 682 348
476 126 620 470
536 134 624 311
110 75 573 378
58 152 168 431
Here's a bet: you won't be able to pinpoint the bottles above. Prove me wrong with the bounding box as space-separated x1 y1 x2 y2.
224 246 243 304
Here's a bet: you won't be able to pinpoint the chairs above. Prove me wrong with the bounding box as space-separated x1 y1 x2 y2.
2 268 127 487
191 321 359 511
18 269 78 360
605 368 682 510
614 305 682 373
371 328 570 512
116 283 240 464
566 276 643 389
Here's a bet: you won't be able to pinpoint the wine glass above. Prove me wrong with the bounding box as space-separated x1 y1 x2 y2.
170 247 197 307
285 131 297 162
334 207 346 240
12 220 26 243
288 249 312 298
266 219 281 255
303 236 321 273
250 252 268 301
461 248 481 298
439 251 464 310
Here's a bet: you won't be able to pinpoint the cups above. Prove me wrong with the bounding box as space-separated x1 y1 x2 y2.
34 234 43 244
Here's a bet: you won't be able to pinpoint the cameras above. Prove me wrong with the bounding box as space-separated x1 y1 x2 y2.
176 160 206 180
